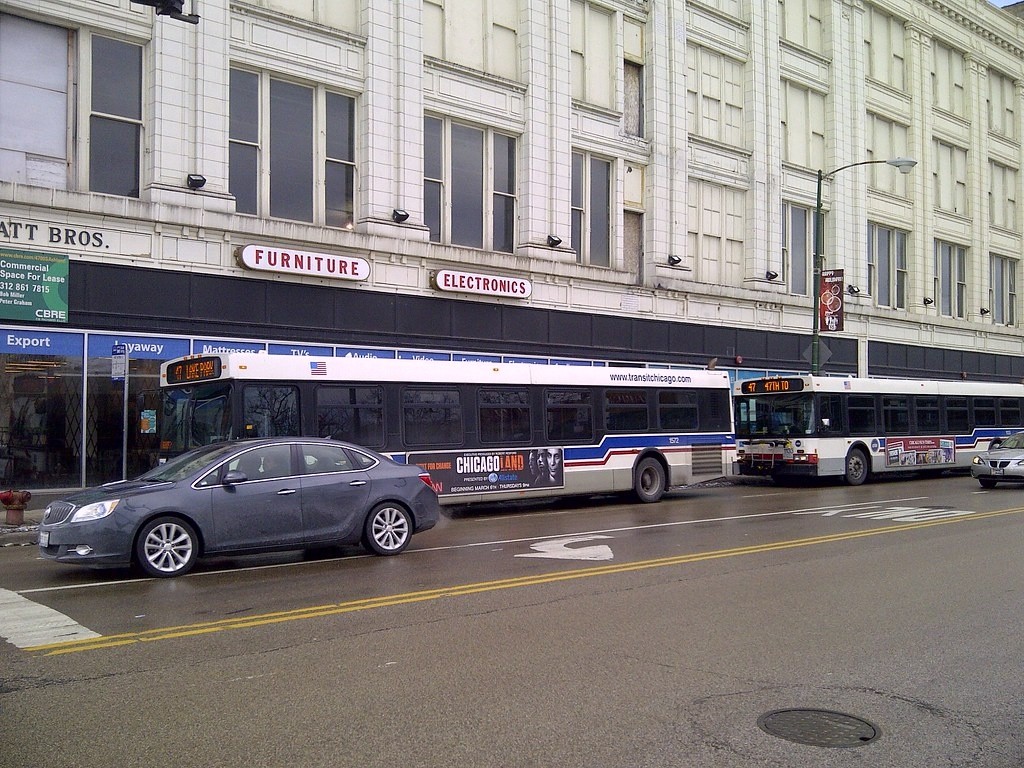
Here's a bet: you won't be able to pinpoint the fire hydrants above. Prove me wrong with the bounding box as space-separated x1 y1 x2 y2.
0 489 34 527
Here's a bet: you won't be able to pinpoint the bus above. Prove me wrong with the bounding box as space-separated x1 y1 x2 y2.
154 352 743 511
729 371 1024 487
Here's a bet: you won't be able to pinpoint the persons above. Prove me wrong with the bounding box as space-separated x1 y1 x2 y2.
239 452 284 478
528 449 564 486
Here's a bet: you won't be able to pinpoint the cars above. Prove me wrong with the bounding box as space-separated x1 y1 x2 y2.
970 430 1024 488
33 437 440 579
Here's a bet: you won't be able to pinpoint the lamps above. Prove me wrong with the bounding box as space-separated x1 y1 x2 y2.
981 308 990 315
767 271 779 280
669 256 681 265
925 298 933 305
393 209 409 222
187 174 206 189
548 236 562 247
848 285 860 294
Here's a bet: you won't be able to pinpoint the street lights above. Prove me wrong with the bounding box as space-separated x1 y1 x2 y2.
811 158 919 373
